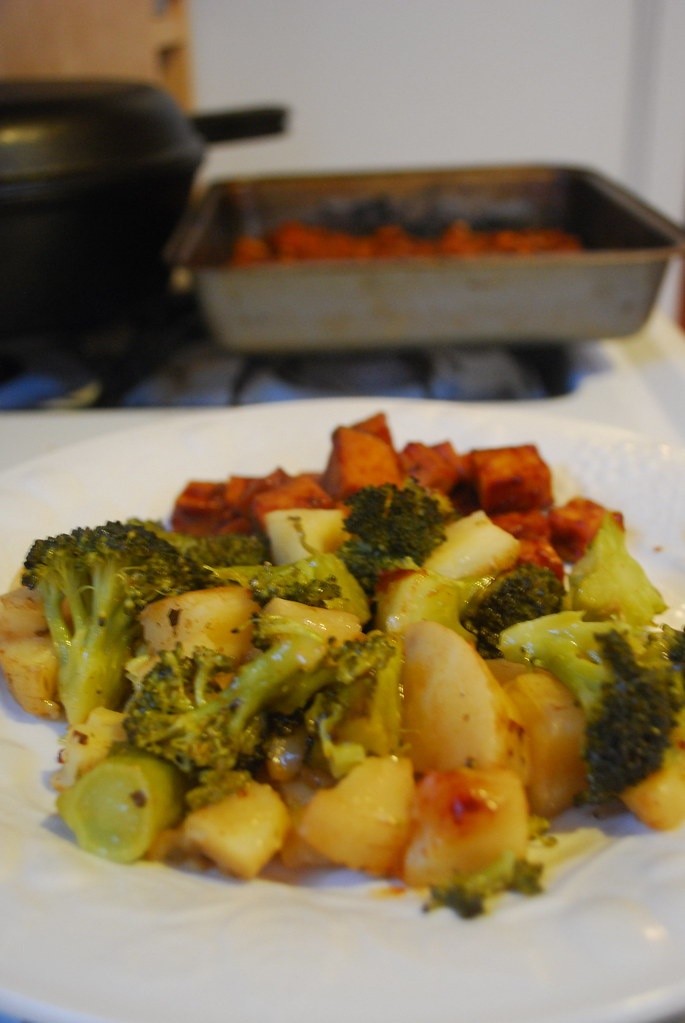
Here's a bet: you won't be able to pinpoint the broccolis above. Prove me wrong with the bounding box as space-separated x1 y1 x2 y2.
24 482 684 796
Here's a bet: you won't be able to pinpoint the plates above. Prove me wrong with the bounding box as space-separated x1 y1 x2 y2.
0 396 685 1022
170 157 683 350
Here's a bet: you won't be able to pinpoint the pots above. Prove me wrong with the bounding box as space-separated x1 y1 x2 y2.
0 77 288 385
